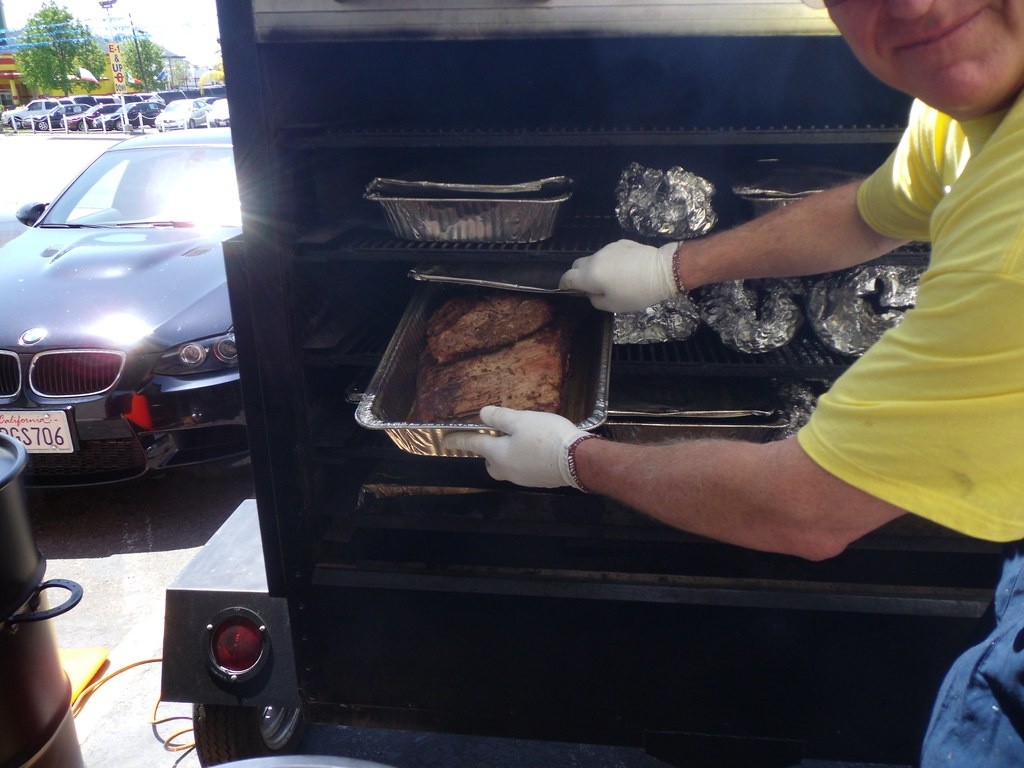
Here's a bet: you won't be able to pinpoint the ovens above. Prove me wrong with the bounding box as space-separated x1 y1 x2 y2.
161 0 1024 768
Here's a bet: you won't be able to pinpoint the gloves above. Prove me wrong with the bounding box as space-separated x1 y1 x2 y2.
444 406 608 495
559 239 683 314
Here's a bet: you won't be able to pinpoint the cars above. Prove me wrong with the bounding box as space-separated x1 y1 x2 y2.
207 99 229 129
67 85 226 100
0 128 245 492
154 99 208 131
22 103 90 130
60 105 126 130
92 102 167 131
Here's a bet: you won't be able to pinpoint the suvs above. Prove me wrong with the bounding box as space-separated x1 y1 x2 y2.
1 99 74 129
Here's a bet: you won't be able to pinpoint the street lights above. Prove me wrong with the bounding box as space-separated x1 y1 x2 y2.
159 55 186 87
133 30 149 94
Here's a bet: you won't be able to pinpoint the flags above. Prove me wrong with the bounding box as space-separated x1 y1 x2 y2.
79 67 100 86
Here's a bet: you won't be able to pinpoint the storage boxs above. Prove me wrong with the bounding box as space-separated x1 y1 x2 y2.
359 171 578 242
352 271 622 466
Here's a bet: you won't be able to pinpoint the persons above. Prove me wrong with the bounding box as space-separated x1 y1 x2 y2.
442 0 1022 768
